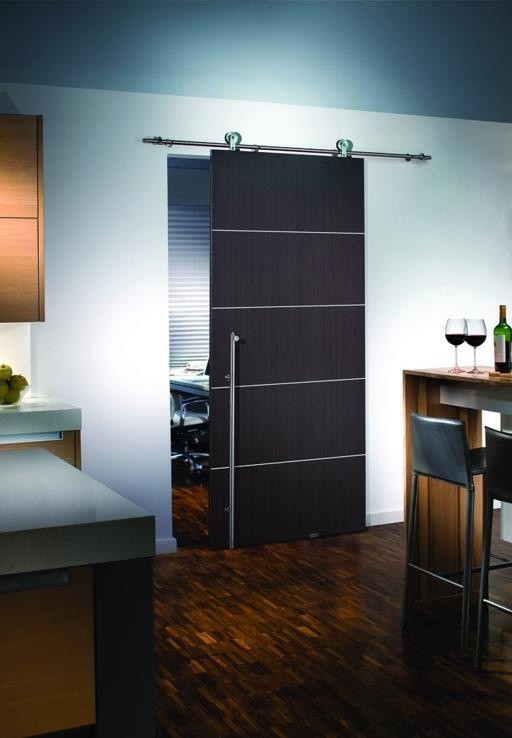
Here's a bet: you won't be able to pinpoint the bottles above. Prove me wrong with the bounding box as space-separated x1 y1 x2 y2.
493 303 511 374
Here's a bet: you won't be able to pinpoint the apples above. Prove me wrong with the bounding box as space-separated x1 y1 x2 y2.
0 364 28 405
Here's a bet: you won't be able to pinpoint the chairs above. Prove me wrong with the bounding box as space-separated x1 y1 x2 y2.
170 393 211 480
399 411 512 673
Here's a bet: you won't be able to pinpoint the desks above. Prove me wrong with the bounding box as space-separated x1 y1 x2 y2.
169 366 212 399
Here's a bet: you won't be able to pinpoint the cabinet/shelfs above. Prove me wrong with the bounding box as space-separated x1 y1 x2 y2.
1 113 45 323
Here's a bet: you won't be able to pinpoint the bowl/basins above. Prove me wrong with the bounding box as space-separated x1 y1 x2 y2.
0 384 32 408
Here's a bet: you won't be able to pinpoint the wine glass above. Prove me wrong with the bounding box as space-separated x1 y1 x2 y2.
445 318 487 374
